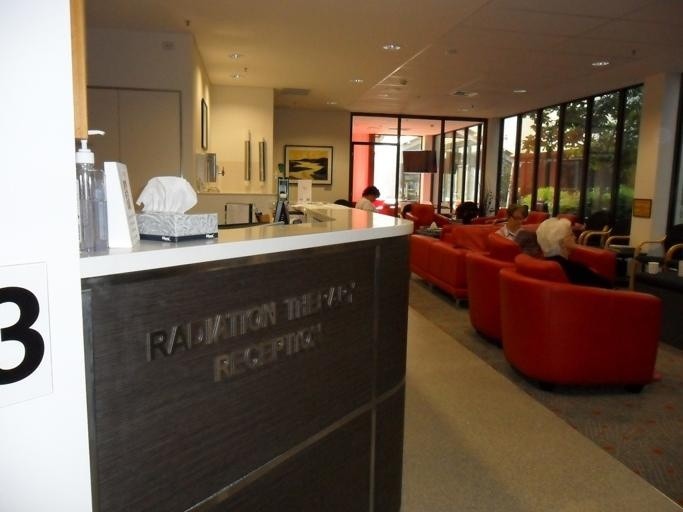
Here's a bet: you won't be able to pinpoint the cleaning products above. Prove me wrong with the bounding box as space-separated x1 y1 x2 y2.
75 130 108 255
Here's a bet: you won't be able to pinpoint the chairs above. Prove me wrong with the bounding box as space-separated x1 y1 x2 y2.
627 225 683 289
406 211 448 234
582 221 631 250
500 267 661 394
470 208 581 243
467 253 514 348
577 217 609 248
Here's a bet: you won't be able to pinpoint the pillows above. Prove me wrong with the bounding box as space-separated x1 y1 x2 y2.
410 204 434 226
489 233 522 264
515 254 568 283
442 221 500 252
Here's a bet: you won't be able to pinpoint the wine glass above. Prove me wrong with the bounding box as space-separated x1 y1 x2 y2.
255 209 262 225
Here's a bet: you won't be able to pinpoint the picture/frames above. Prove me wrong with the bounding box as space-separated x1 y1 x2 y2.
284 145 333 186
633 199 652 218
201 98 208 151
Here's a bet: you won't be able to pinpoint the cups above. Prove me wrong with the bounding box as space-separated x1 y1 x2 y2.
648 262 659 275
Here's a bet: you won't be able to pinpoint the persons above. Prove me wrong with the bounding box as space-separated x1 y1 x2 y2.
356 186 380 211
536 217 615 289
495 204 525 241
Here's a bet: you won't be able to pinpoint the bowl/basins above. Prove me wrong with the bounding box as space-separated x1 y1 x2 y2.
260 214 270 224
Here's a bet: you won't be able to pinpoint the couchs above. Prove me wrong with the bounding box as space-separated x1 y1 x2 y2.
411 233 468 307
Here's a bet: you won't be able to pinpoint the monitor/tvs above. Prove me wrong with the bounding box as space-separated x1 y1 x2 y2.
403 151 437 172
